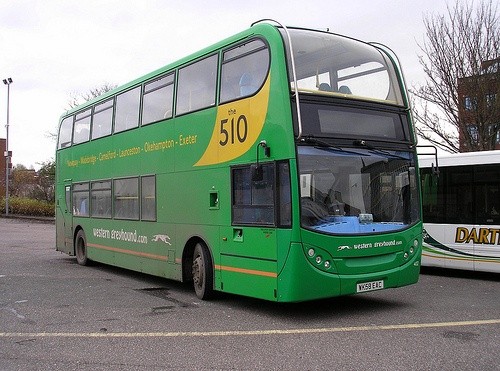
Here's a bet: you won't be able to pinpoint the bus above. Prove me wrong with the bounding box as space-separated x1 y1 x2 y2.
413 150 500 276
56 21 423 303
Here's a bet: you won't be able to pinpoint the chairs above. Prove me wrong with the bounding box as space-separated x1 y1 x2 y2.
238 72 352 96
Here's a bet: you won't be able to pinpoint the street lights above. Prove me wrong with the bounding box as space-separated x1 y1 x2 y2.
2 77 12 216
